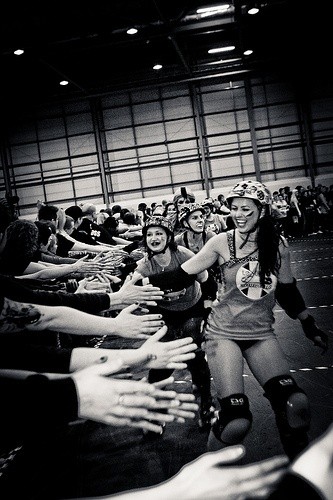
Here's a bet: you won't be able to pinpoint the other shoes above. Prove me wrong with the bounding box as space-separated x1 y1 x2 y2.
140 419 165 436
197 401 219 429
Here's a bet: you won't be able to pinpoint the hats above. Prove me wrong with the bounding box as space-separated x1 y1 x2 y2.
33 221 51 238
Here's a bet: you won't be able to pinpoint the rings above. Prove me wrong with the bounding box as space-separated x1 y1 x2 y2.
118 395 123 405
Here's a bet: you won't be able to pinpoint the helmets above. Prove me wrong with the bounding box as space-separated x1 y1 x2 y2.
177 203 205 224
200 199 212 206
225 180 271 215
141 216 174 235
172 187 194 205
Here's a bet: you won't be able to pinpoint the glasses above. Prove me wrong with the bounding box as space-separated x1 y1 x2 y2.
177 200 190 204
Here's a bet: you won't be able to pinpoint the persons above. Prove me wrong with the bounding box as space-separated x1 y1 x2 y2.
264 427 333 500
174 204 217 311
0 184 333 500
135 180 328 463
168 187 195 231
200 200 227 235
135 215 216 442
